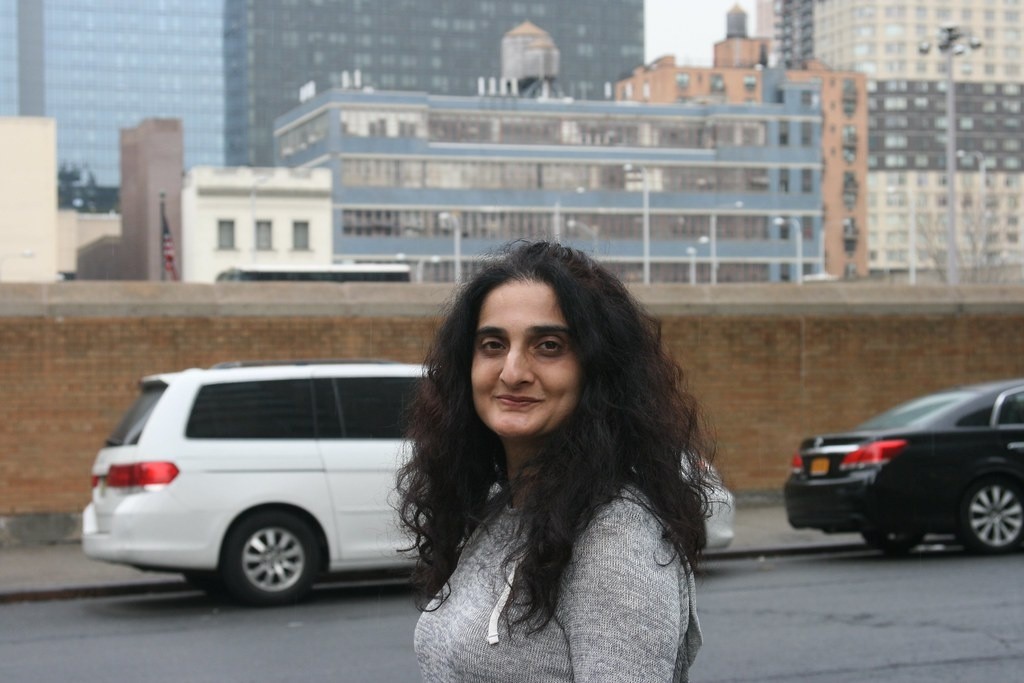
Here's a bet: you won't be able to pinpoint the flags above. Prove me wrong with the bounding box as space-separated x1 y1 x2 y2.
163 215 180 279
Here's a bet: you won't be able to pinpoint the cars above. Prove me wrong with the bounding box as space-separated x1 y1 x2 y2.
780 376 1024 557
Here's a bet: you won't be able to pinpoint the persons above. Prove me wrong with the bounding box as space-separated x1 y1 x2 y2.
392 236 715 683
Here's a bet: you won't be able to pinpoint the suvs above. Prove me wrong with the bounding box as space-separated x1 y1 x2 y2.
79 358 737 607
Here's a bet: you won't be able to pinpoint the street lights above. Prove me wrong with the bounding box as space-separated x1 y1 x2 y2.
918 21 984 285
773 216 803 285
566 219 600 257
623 162 649 286
438 212 462 285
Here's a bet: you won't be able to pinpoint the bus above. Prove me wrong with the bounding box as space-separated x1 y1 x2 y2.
216 263 410 286
